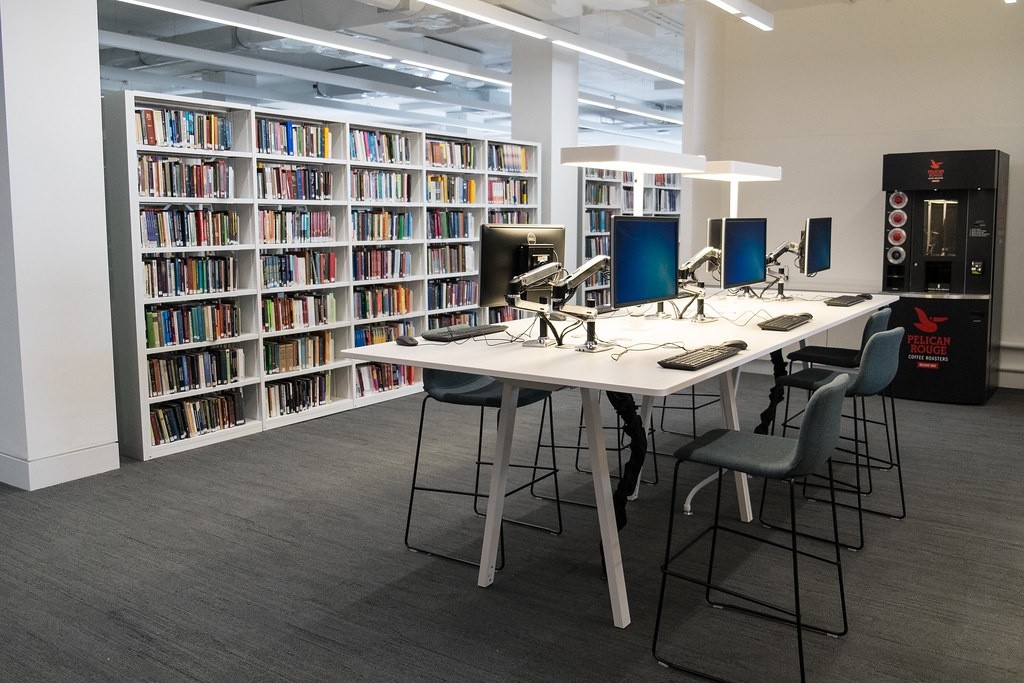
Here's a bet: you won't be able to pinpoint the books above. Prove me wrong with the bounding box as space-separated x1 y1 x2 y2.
488 144 530 224
349 129 415 397
135 109 245 446
489 307 513 325
254 117 336 418
426 141 479 330
585 168 676 307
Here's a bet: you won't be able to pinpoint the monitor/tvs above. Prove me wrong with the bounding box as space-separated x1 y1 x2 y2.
478 221 566 307
609 214 678 311
798 217 831 276
705 218 766 290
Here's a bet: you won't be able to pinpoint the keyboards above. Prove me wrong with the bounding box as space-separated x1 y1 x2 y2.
757 315 809 332
657 345 741 371
421 324 508 342
824 295 866 307
595 304 617 315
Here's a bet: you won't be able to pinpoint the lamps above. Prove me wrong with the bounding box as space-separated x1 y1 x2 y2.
418 0 685 85
709 0 775 31
118 0 512 88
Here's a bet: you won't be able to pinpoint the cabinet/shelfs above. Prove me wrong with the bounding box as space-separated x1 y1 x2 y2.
102 89 542 463
576 167 681 307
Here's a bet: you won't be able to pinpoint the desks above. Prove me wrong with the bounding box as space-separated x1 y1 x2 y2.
339 290 899 629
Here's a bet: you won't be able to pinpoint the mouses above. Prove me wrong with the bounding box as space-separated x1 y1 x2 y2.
720 340 748 351
793 312 813 320
857 294 873 300
395 336 418 346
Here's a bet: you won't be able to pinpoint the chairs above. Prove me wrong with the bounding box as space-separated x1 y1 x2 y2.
404 369 563 572
757 325 906 552
530 385 720 508
650 372 849 683
781 308 892 496
